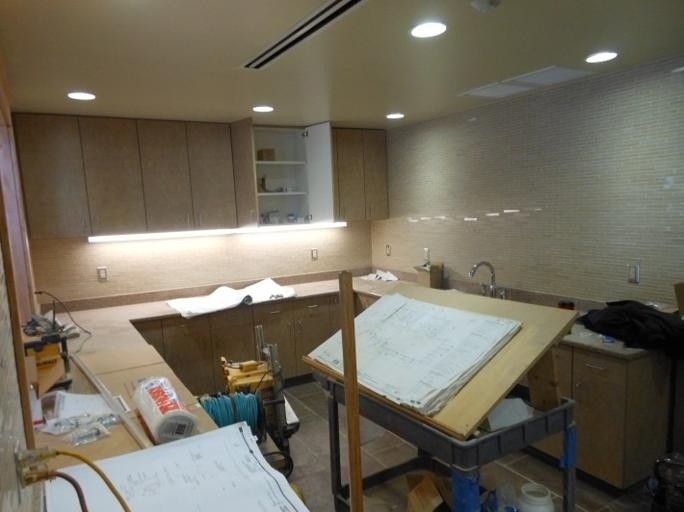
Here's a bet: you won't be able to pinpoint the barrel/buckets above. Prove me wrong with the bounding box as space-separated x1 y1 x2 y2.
516 482 556 512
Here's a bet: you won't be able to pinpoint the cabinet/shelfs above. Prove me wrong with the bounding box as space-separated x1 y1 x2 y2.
230 115 340 228
572 351 674 498
333 127 387 222
12 111 238 239
133 293 333 398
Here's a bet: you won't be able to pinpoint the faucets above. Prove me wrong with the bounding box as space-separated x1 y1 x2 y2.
468 261 497 298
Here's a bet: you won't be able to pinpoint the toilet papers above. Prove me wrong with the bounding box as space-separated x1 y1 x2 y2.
132 375 199 444
